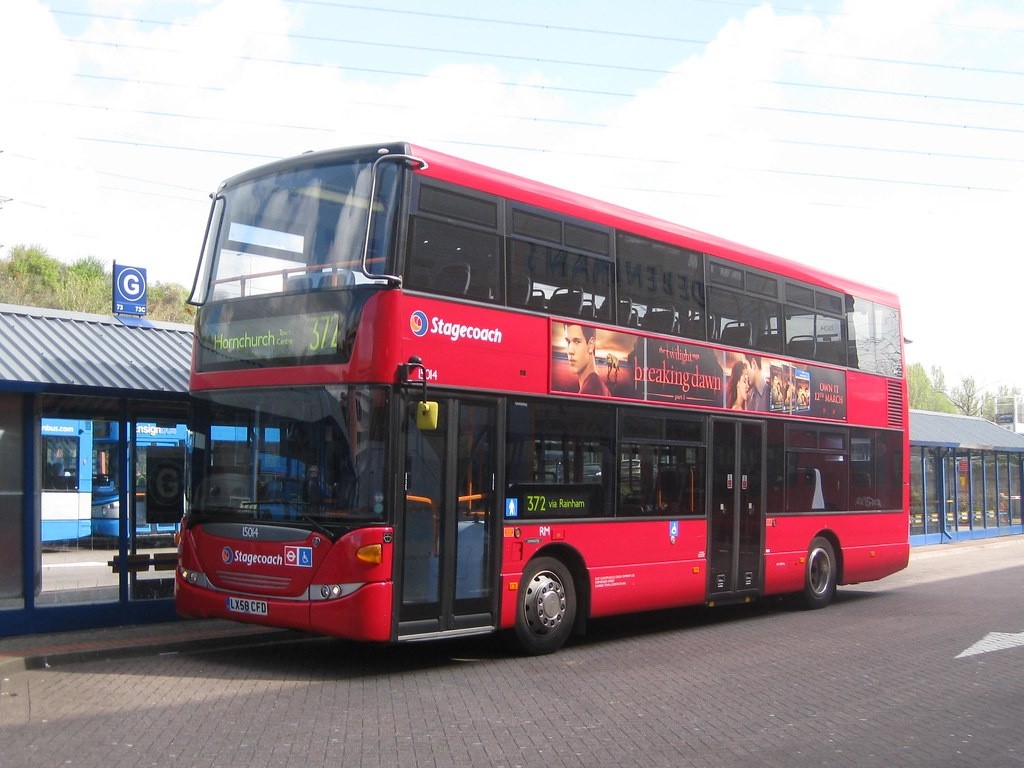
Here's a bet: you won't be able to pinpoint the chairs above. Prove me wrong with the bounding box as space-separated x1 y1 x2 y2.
285 261 870 514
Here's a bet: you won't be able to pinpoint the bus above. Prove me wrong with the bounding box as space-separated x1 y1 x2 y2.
171 142 914 654
186 426 290 509
40 419 93 545
95 423 194 540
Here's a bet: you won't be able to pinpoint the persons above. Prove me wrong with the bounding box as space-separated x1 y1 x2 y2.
744 354 771 412
768 374 813 414
563 323 612 397
726 360 749 410
